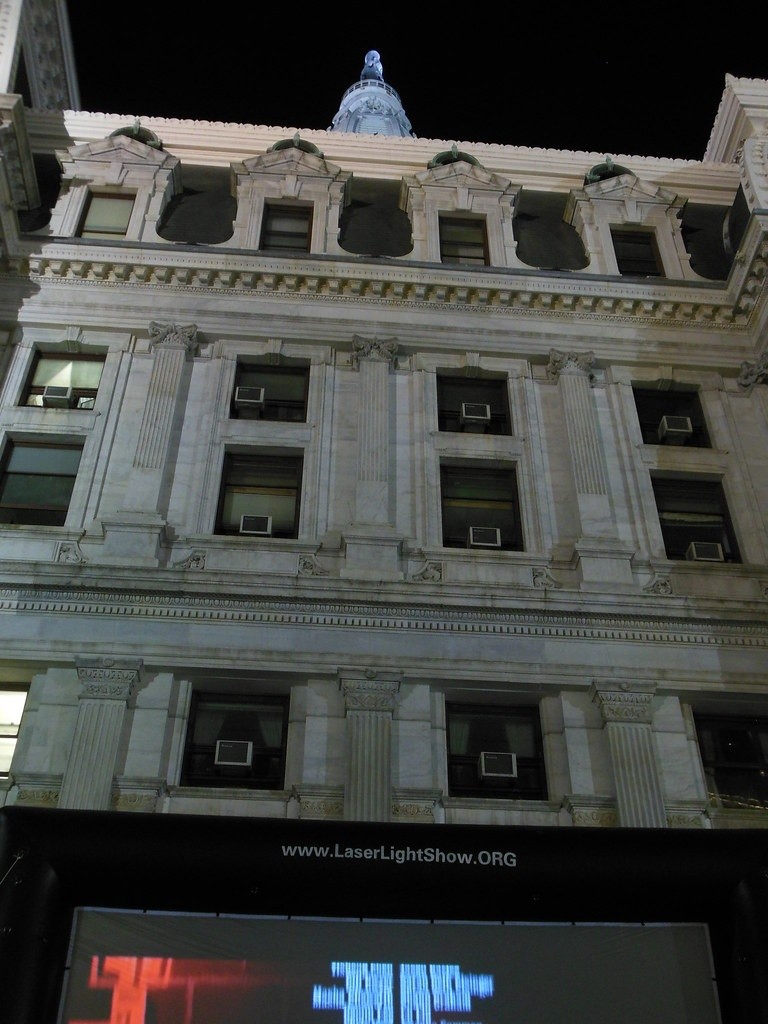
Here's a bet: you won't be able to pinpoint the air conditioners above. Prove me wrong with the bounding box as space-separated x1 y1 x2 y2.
658 416 692 444
478 752 517 782
239 515 273 536
215 741 253 769
42 385 73 408
465 526 501 550
460 402 491 423
233 386 265 410
686 542 725 562
